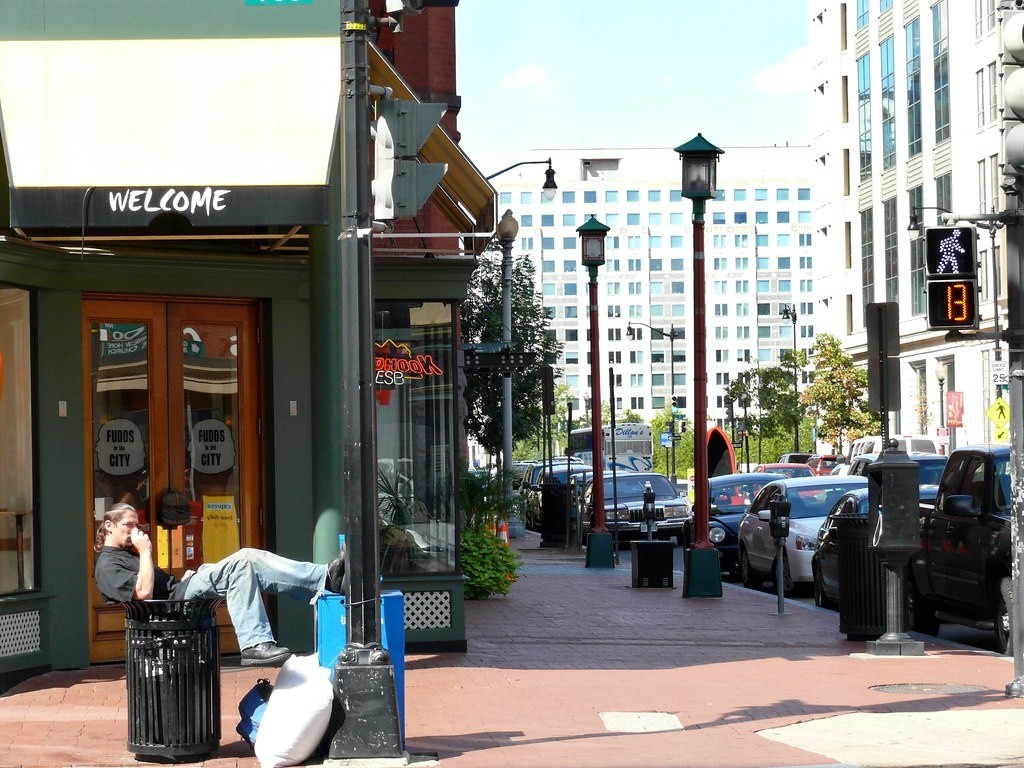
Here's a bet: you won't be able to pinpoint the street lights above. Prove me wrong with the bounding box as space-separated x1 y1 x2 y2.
575 214 616 569
782 304 800 454
626 321 679 483
585 394 591 429
674 132 726 597
499 209 518 493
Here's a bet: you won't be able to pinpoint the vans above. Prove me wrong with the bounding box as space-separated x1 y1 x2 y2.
848 433 943 459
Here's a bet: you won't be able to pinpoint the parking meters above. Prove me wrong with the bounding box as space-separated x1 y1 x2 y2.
642 487 656 540
768 492 792 617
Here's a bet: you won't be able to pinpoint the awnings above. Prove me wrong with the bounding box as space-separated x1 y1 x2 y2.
364 40 498 256
0 37 342 254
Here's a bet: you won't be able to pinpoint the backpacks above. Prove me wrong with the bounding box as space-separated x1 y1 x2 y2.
234 678 275 748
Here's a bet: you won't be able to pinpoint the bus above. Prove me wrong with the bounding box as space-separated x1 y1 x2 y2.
569 423 653 473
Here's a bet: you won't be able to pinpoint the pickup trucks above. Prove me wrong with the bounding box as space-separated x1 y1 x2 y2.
898 443 1016 654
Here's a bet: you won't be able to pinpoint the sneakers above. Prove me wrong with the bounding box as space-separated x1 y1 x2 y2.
324 550 347 594
240 642 290 665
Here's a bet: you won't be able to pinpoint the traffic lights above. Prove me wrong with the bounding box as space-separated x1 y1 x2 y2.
672 396 677 407
681 422 686 433
925 226 980 327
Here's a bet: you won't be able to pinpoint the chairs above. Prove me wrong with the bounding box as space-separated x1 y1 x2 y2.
825 491 844 510
604 484 614 498
789 497 807 516
713 492 733 506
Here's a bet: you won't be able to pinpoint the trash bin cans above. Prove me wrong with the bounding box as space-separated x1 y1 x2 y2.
538 483 577 548
120 595 224 765
828 510 911 642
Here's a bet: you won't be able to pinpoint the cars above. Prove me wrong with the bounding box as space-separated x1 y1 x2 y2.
753 450 951 506
738 475 871 595
576 470 691 544
682 470 790 580
811 482 943 612
499 455 629 520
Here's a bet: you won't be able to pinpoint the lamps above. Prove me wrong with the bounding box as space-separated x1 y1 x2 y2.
485 157 558 201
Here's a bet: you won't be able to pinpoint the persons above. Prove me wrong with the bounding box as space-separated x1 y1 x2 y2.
94 502 347 665
473 464 503 500
